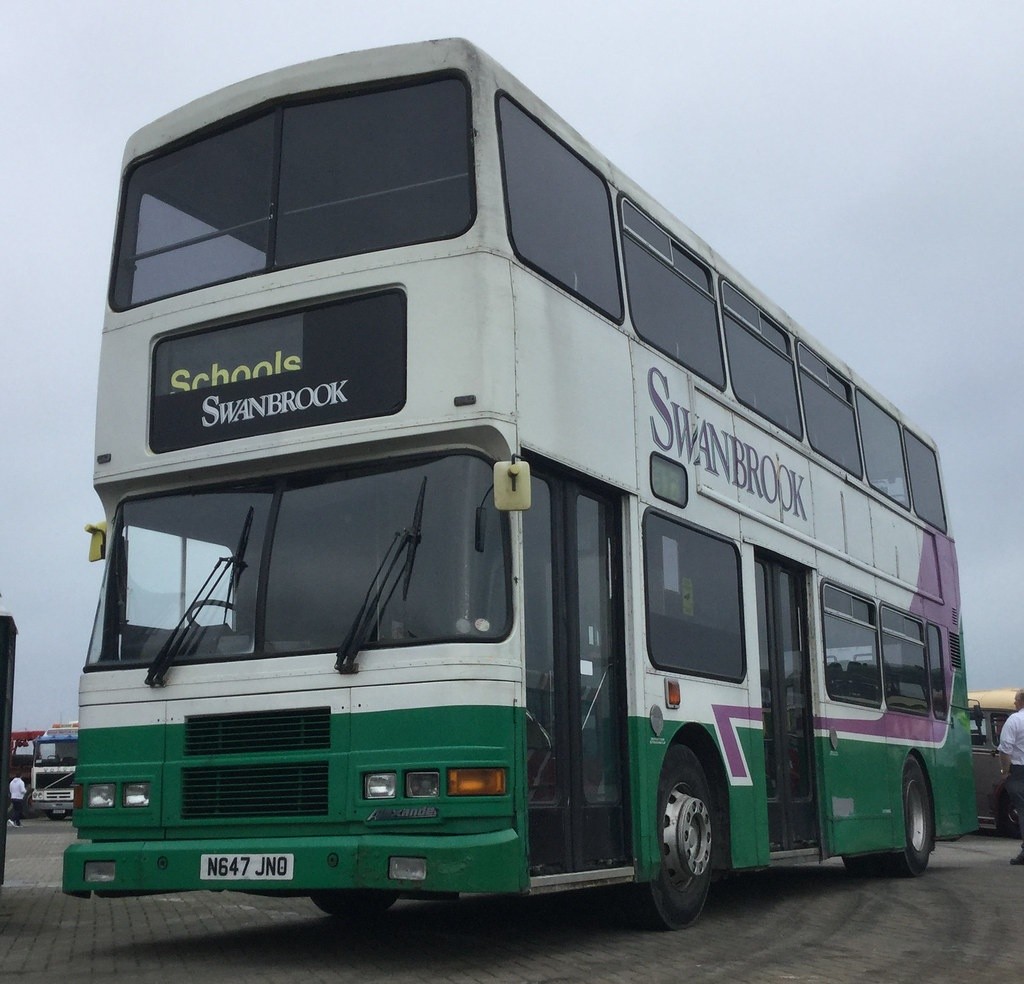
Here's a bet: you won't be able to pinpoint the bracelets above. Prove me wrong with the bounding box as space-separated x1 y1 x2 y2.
1000 770 1003 774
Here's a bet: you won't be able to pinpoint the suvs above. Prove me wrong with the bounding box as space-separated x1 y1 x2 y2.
969 707 1023 837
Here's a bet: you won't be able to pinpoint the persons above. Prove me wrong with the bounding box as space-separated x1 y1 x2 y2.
997 690 1024 865
8 771 26 828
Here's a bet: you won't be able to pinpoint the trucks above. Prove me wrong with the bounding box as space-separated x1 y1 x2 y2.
8 722 80 821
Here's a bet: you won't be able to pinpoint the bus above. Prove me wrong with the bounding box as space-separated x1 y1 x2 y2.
62 34 978 932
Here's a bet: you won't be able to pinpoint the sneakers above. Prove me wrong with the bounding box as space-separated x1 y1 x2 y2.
1010 851 1024 865
8 819 23 828
